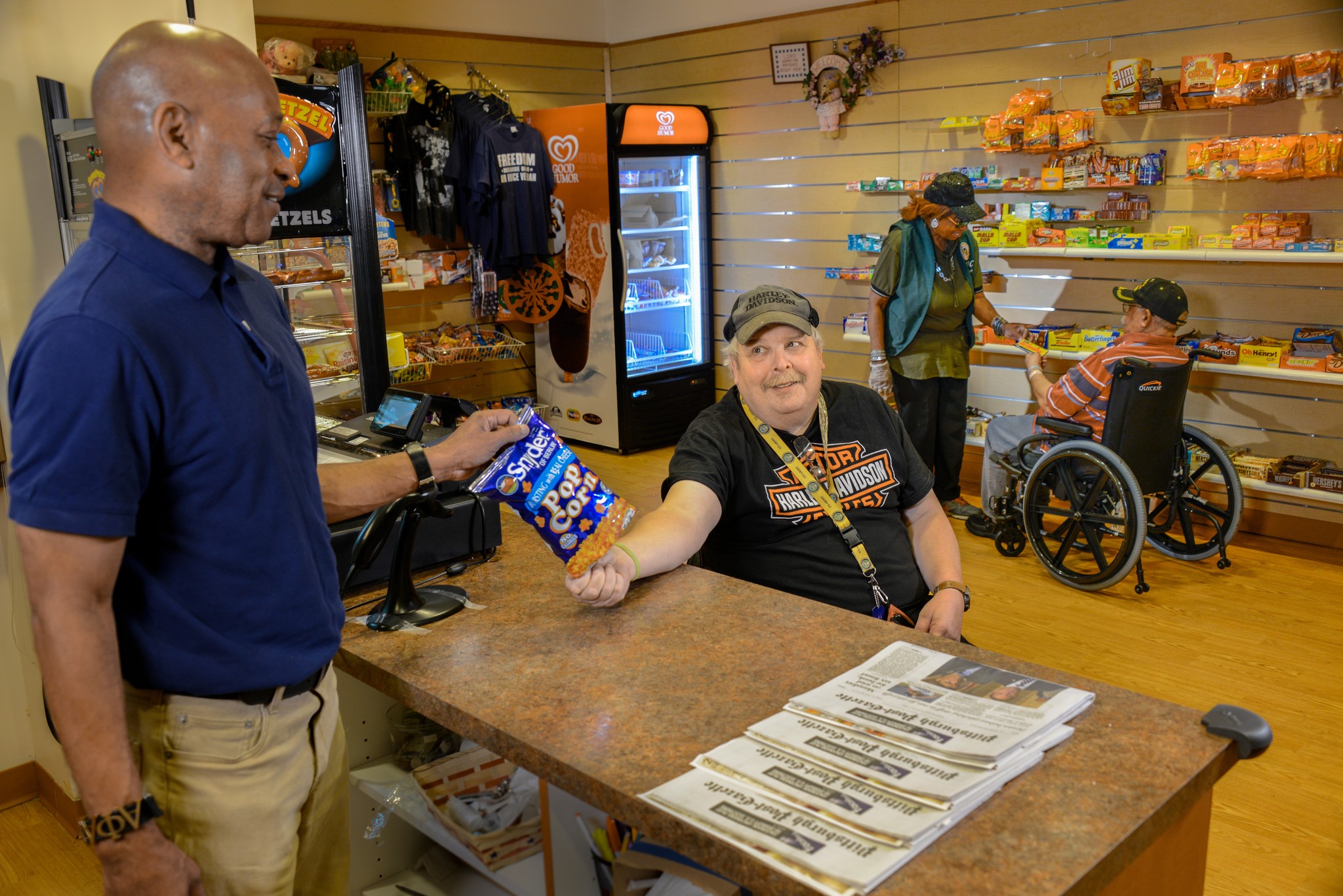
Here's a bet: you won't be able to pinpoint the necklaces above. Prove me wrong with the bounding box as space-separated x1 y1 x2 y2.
936 255 955 283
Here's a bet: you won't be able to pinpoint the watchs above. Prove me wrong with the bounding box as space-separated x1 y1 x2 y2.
933 580 971 612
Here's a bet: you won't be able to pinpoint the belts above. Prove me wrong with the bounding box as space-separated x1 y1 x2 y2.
160 660 336 704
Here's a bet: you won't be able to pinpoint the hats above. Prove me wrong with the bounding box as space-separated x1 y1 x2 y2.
1112 277 1189 326
923 172 987 223
723 285 820 344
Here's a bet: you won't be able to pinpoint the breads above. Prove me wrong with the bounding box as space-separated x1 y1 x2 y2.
305 363 341 380
264 267 345 286
291 324 295 332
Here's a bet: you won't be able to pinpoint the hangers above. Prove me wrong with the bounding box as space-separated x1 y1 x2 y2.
469 68 512 124
404 65 447 106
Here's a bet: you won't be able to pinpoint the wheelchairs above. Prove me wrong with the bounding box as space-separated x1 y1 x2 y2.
988 347 1244 596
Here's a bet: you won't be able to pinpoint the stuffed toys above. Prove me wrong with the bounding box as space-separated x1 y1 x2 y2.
257 40 304 76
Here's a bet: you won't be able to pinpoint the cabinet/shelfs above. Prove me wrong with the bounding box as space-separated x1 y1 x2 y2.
837 104 1343 530
227 166 549 430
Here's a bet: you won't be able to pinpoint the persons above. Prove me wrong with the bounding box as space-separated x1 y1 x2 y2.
565 284 978 648
964 276 1189 540
867 171 1036 519
5 18 530 895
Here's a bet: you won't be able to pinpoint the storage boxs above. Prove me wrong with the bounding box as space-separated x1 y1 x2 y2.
1180 50 1232 97
838 163 1343 498
1138 78 1180 112
1106 53 1155 98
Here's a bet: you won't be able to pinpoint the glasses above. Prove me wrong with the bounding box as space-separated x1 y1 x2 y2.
1123 302 1155 318
947 216 969 228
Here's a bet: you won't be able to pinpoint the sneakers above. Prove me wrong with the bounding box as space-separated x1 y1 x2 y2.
1015 503 1043 534
941 496 982 520
965 514 1024 544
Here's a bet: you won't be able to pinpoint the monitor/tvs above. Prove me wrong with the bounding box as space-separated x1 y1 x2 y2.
370 388 431 445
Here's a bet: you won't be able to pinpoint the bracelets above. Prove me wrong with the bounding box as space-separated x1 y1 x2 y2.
870 350 887 362
77 792 166 846
993 316 1008 337
399 439 436 493
1027 365 1043 380
613 542 640 582
